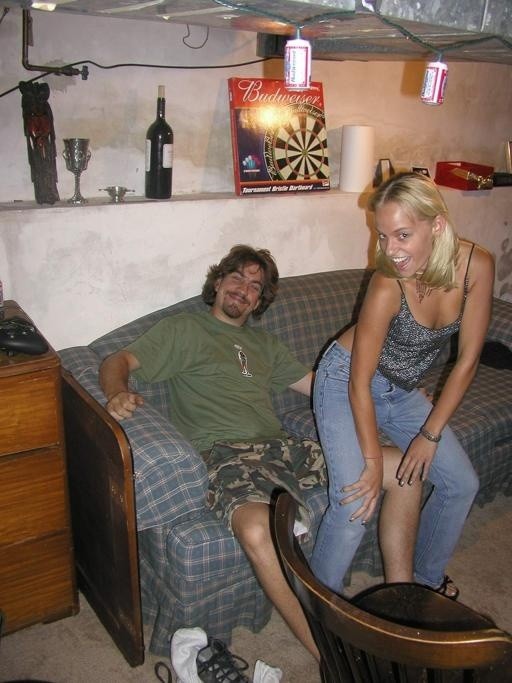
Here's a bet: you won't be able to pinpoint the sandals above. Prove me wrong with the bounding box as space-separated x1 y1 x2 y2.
436 576 460 601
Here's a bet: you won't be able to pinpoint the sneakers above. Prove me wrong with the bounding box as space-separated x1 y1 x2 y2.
170 626 250 683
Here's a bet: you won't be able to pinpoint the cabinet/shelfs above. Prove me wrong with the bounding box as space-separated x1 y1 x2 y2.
0 296 79 636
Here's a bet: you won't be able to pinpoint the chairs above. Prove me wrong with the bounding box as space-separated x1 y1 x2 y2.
271 492 510 680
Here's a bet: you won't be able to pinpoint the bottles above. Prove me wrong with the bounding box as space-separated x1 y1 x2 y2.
0 280 3 307
144 84 175 200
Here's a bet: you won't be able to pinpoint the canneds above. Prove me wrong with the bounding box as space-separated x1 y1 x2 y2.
283 39 312 92
420 61 448 106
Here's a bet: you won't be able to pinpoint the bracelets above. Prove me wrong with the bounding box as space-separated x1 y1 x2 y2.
420 426 442 441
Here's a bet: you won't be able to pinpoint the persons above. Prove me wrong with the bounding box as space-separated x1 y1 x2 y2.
100 247 422 664
310 175 495 602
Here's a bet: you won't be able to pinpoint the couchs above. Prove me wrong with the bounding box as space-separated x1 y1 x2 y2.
55 266 510 666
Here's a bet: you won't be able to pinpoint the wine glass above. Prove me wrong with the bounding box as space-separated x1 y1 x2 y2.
62 135 90 205
98 185 136 203
